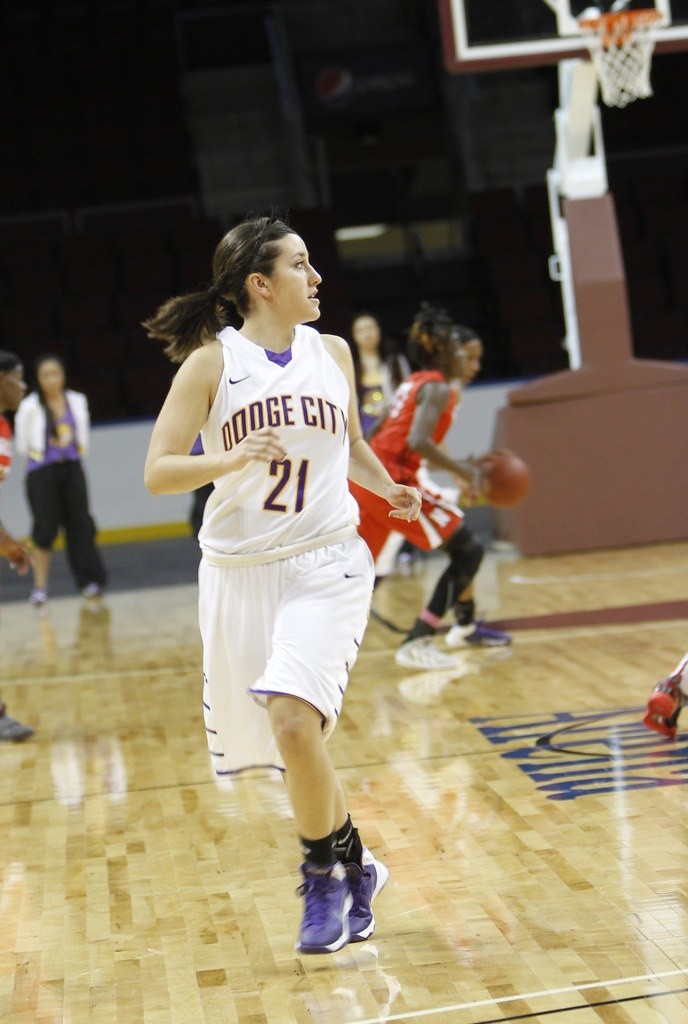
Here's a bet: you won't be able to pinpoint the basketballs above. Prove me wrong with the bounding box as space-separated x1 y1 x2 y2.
477 449 533 507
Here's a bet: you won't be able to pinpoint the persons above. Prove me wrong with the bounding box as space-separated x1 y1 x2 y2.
13 351 110 605
643 651 688 741
0 353 33 741
143 216 423 953
347 307 512 670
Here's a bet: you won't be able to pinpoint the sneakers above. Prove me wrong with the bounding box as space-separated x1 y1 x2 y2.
398 638 454 669
643 678 683 739
347 846 389 943
444 619 512 647
0 716 36 741
294 859 353 953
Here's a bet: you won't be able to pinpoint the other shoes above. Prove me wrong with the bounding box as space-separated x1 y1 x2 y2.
397 551 413 575
31 589 47 607
82 583 103 598
